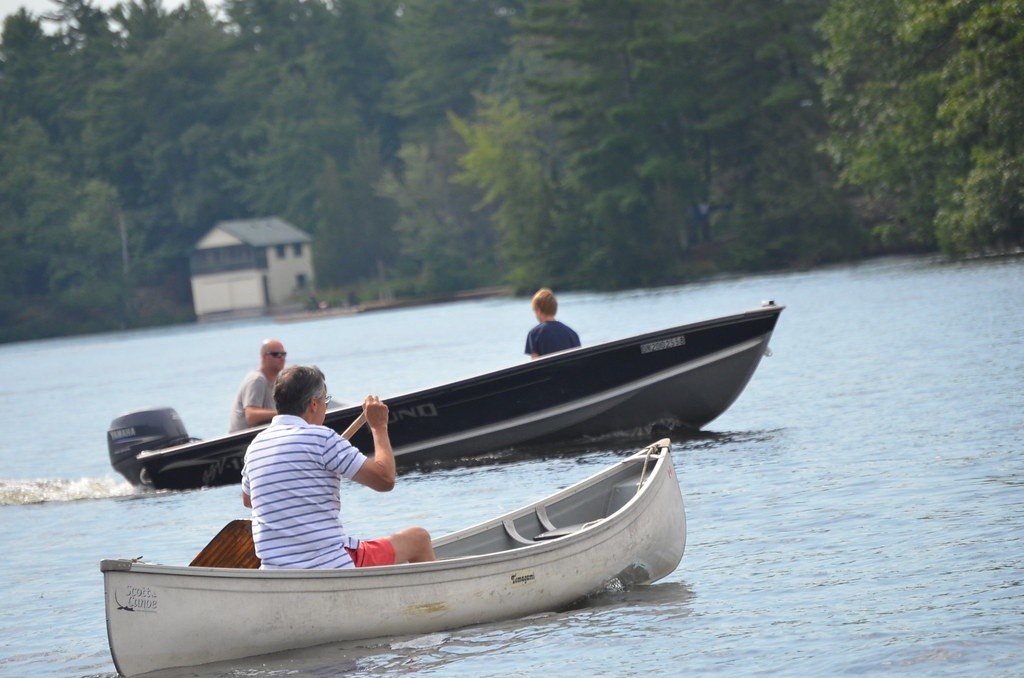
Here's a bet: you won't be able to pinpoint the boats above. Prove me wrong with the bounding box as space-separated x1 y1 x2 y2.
99 435 687 678
106 298 787 490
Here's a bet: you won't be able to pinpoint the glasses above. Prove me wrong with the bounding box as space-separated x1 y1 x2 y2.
267 351 286 358
307 395 331 406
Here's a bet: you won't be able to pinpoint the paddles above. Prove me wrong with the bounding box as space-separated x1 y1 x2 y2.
188 411 369 567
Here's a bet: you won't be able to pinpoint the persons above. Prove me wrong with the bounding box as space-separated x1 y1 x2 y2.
240 367 435 569
524 288 580 358
229 339 287 433
